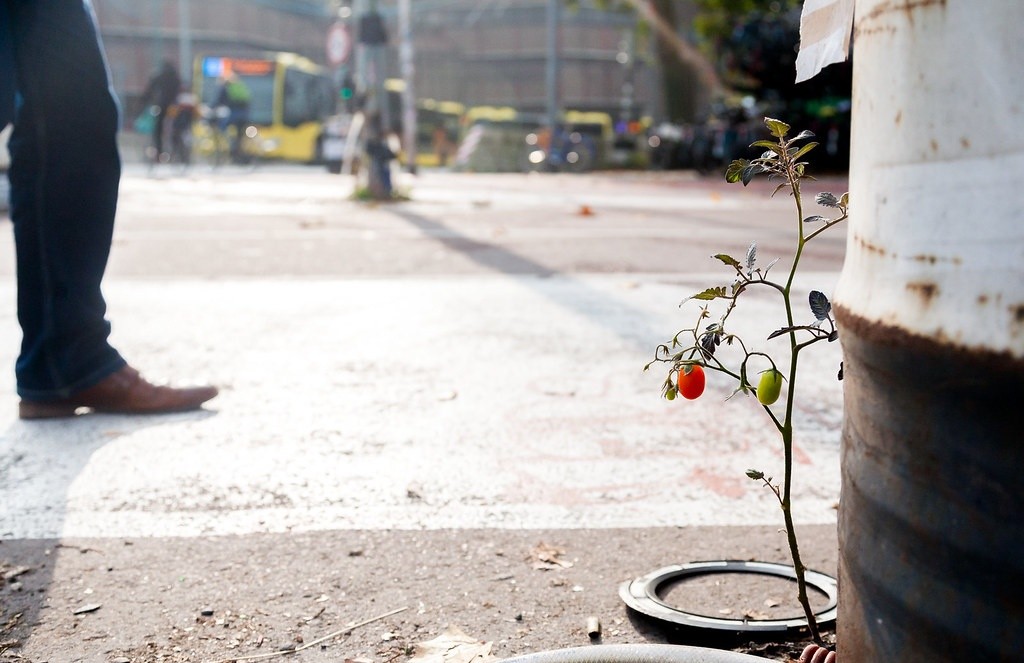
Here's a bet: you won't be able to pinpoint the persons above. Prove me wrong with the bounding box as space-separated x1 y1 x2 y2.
129 50 251 167
345 8 394 203
1 0 219 419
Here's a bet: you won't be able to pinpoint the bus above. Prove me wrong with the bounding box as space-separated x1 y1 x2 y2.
191 50 652 171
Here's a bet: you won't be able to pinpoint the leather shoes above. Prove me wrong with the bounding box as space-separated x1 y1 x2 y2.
19 363 219 418
